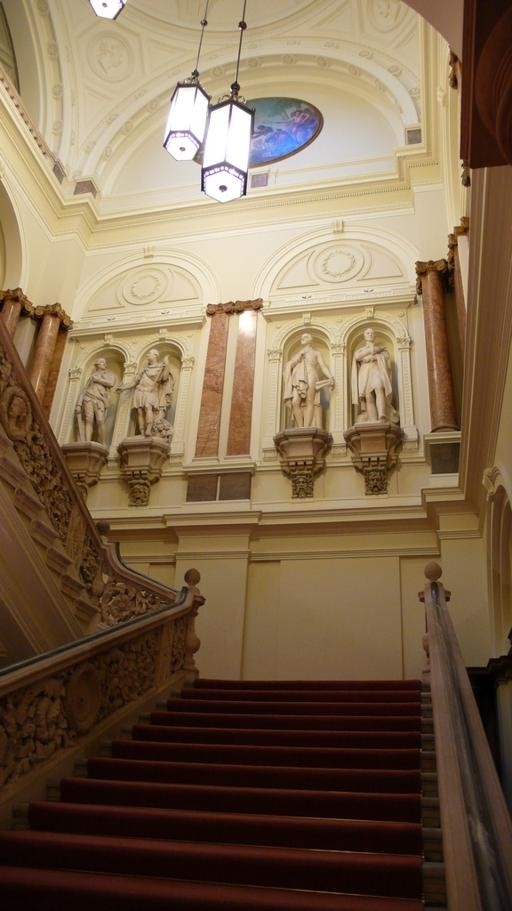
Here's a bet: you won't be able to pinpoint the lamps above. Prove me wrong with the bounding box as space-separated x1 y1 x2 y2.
162 0 212 161
200 0 255 204
88 0 126 21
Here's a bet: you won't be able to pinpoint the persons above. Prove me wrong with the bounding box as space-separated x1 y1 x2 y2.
354 328 392 422
114 349 170 437
251 122 274 145
263 128 292 155
76 358 117 443
283 333 337 429
283 109 310 135
